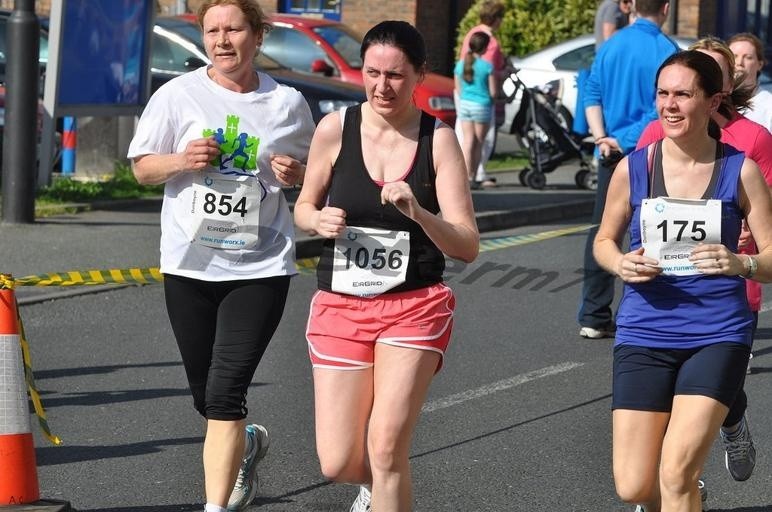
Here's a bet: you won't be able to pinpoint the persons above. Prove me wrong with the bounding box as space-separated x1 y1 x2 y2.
127 0 316 512
293 20 479 512
727 30 772 135
454 30 504 190
594 0 632 52
590 48 772 510
459 2 519 188
636 35 772 483
578 0 684 340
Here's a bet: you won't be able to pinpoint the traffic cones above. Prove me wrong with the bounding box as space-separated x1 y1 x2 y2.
0 271 72 512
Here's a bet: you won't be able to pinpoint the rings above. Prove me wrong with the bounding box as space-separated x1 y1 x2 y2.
633 262 638 273
716 259 722 267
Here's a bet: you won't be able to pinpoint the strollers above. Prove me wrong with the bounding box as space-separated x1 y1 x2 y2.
504 67 599 191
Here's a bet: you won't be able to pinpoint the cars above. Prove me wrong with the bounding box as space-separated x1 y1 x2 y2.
501 30 772 141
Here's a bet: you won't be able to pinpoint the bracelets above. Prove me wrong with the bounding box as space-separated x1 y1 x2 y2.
739 253 758 280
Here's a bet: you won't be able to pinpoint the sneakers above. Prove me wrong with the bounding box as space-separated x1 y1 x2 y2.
579 319 617 339
720 415 756 482
226 424 270 512
349 484 372 512
